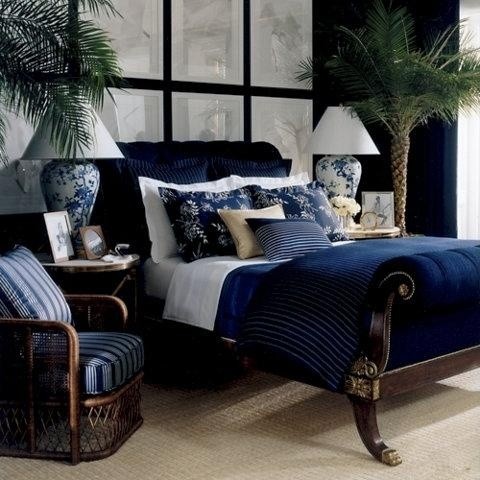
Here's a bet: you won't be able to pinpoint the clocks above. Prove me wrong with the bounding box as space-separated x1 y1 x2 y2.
361 211 378 230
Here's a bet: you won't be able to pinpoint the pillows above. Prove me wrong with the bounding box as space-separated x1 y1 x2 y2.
117 156 352 263
0 244 75 351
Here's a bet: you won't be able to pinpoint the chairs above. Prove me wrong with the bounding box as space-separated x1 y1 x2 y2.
0 292 146 466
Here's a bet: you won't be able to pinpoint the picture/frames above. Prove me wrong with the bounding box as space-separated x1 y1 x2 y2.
361 190 395 230
78 225 108 260
44 210 75 263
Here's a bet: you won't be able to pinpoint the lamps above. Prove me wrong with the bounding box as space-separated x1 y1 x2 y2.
19 96 126 259
302 106 381 225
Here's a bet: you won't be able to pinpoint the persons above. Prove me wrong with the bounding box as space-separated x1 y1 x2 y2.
56 221 68 250
372 196 387 225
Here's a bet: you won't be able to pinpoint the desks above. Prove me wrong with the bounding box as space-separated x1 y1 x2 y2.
32 253 141 331
345 224 402 240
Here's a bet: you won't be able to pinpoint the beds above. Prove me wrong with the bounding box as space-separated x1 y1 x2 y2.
84 140 480 467
